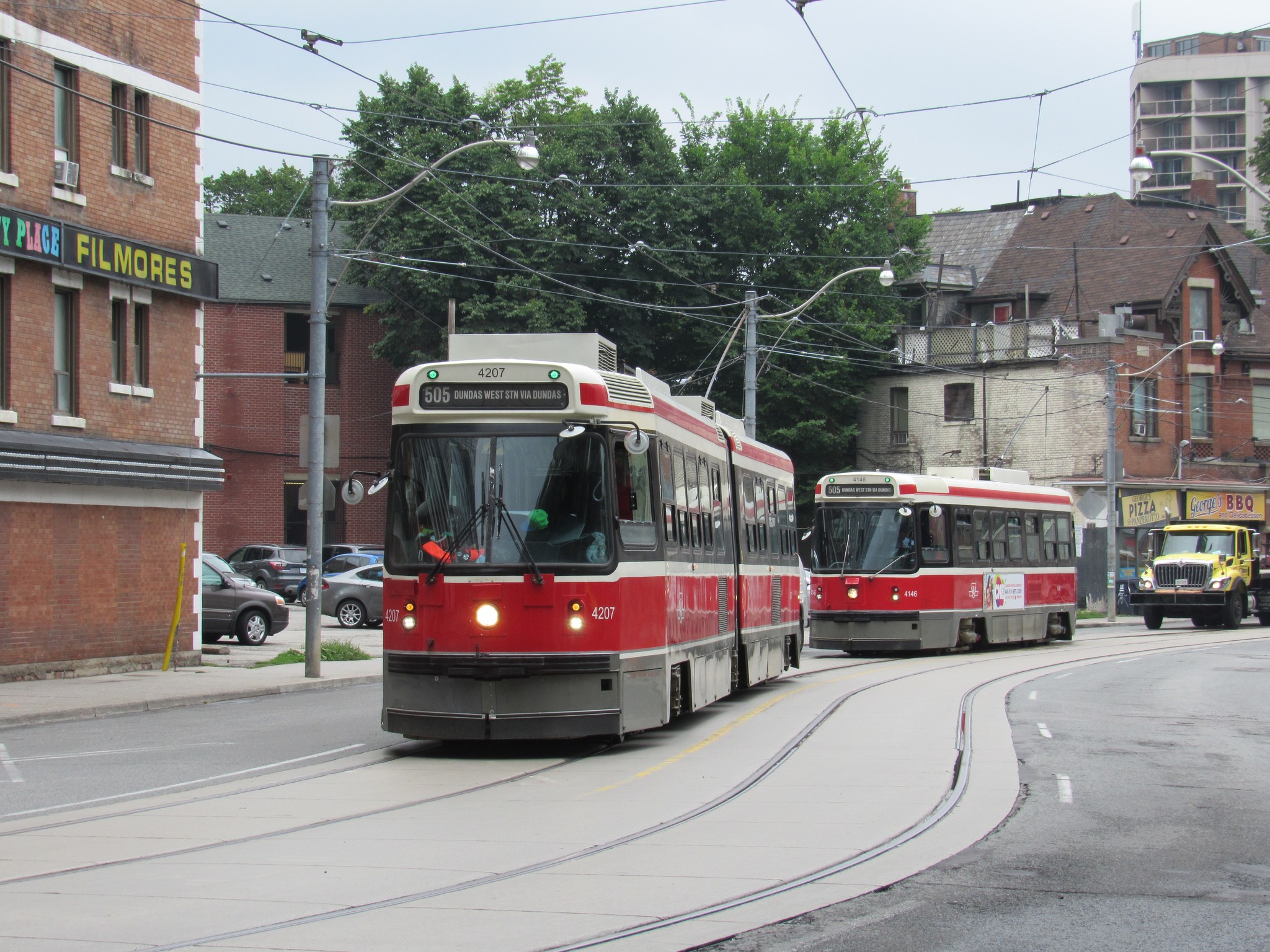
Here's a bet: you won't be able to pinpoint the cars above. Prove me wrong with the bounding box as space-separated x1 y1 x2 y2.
202 558 290 646
321 563 384 629
202 551 258 589
296 543 385 607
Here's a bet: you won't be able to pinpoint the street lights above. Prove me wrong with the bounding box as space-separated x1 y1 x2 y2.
1106 335 1227 621
742 258 898 444
303 132 541 679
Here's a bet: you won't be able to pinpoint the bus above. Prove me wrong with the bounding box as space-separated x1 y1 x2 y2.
801 386 1080 661
381 305 805 752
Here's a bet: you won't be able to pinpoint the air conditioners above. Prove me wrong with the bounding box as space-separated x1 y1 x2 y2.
54 160 79 188
1192 330 1205 341
1134 424 1146 435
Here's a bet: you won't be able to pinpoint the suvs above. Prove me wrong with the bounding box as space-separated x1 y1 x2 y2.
222 542 307 604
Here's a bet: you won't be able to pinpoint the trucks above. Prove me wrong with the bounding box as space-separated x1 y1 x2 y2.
1129 506 1269 630
